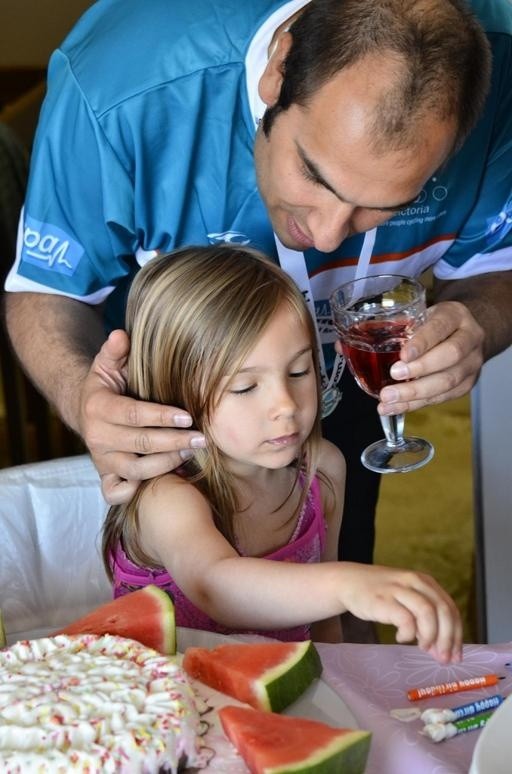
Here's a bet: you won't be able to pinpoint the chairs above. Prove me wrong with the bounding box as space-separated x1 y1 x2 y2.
1 452 125 642
466 341 511 641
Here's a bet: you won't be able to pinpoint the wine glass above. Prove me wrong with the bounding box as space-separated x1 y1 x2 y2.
332 274 435 476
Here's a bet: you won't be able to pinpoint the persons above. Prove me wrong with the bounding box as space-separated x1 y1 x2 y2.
99 245 466 664
2 0 512 643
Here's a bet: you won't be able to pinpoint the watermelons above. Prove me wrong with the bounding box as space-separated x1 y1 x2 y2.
217 704 372 774
46 584 176 656
182 639 324 715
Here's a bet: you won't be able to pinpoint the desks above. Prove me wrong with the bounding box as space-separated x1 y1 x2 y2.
2 625 508 773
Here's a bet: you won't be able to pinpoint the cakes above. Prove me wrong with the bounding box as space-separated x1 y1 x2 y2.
0 633 201 774
